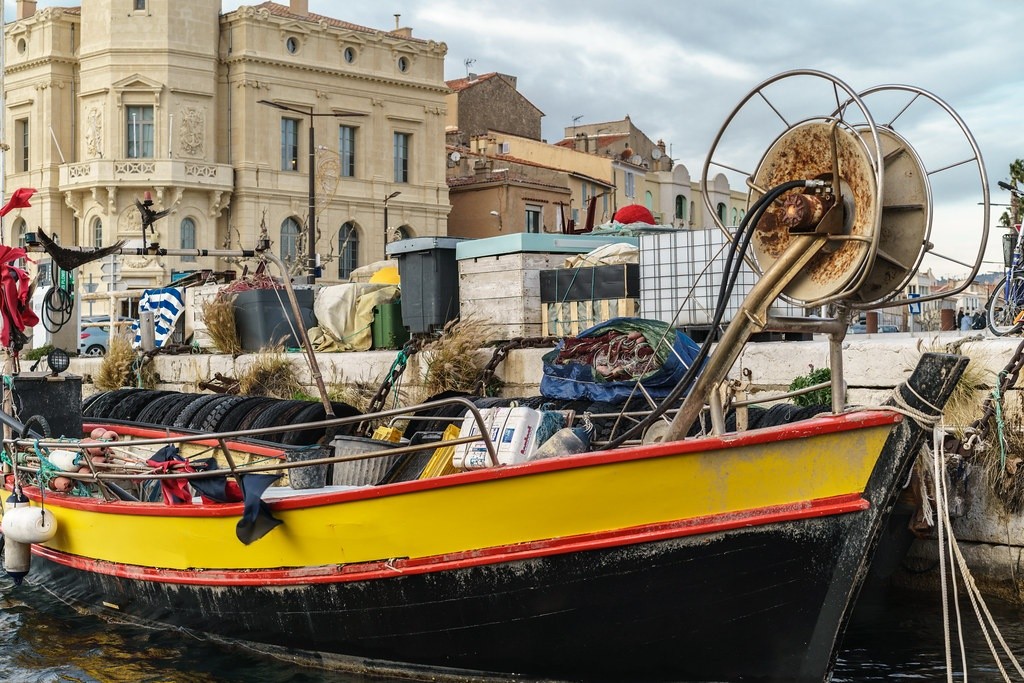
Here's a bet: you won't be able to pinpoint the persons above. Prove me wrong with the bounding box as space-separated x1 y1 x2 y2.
957 306 987 330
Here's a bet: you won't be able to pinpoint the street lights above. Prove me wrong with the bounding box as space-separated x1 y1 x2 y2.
256 99 367 285
383 190 404 260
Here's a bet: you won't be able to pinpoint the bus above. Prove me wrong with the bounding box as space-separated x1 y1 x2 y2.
878 325 899 333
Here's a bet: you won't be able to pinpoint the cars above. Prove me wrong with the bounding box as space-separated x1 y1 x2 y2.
80 314 140 356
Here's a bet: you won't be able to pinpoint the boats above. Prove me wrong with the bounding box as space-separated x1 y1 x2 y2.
0 70 992 683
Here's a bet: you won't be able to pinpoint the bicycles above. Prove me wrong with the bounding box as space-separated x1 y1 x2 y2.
985 182 1024 335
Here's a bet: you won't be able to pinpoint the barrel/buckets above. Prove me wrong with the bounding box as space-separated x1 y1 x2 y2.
332 435 408 486
285 445 331 489
